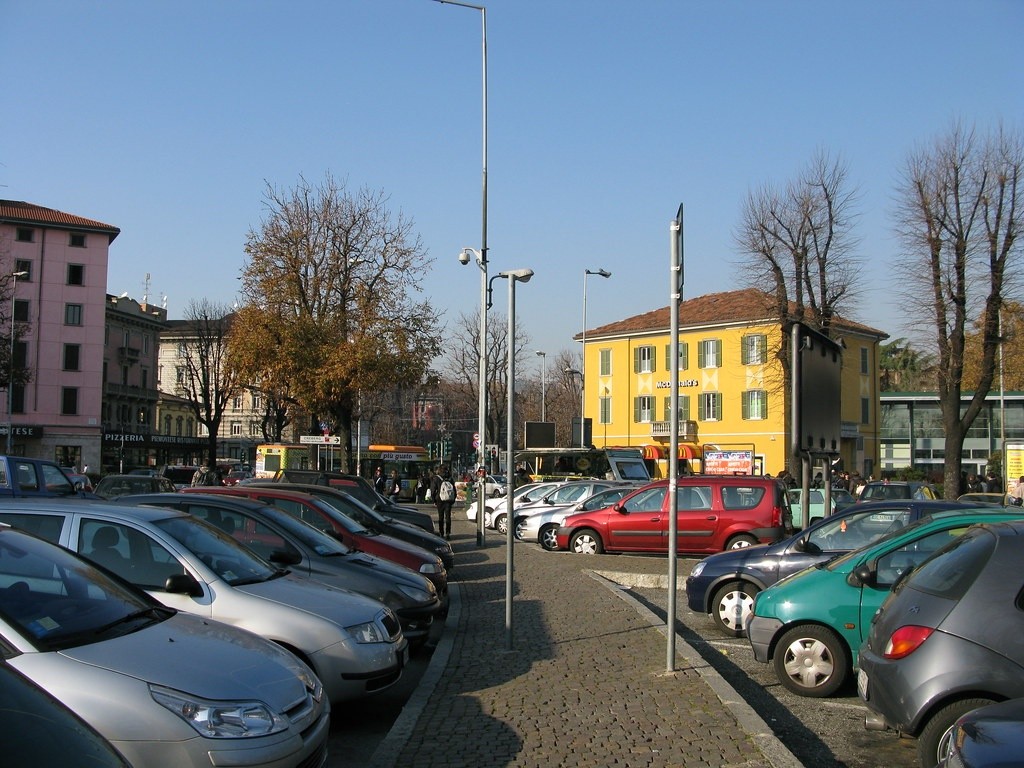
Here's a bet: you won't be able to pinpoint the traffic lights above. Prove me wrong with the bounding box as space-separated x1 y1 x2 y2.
428 441 452 460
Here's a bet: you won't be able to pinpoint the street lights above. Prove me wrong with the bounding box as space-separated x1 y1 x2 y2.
6 271 30 456
536 351 545 422
564 367 584 448
476 268 535 547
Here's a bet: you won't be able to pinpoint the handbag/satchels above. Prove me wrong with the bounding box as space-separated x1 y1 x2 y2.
390 484 399 493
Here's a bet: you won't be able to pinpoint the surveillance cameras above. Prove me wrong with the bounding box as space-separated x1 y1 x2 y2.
459 253 470 265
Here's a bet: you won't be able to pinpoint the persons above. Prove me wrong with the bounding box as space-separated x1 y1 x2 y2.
515 468 533 488
830 469 890 500
244 468 253 480
1012 476 1024 507
960 471 1001 496
82 464 88 474
914 476 936 500
387 470 402 503
72 464 77 474
765 470 797 504
373 466 386 495
464 473 475 485
811 472 825 489
191 458 220 488
431 464 457 540
415 468 434 504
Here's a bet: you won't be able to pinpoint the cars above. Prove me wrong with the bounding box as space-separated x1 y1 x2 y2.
857 521 1024 768
80 493 441 638
476 475 507 498
466 474 1017 555
0 523 333 768
234 469 455 570
687 491 1022 640
0 497 411 701
941 697 1024 768
0 457 255 500
745 509 1023 698
179 486 448 594
0 657 135 768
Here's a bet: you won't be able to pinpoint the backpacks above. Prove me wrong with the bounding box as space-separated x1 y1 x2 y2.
814 479 822 489
436 475 454 501
196 467 213 486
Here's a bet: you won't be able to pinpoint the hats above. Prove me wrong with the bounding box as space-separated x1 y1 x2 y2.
831 470 835 472
377 467 381 470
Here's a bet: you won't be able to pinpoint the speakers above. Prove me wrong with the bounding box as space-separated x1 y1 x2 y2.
572 417 592 448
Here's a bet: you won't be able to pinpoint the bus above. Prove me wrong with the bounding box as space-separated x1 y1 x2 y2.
255 443 452 504
514 448 612 482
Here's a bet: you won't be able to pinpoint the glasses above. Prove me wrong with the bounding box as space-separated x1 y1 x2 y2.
378 470 381 471
